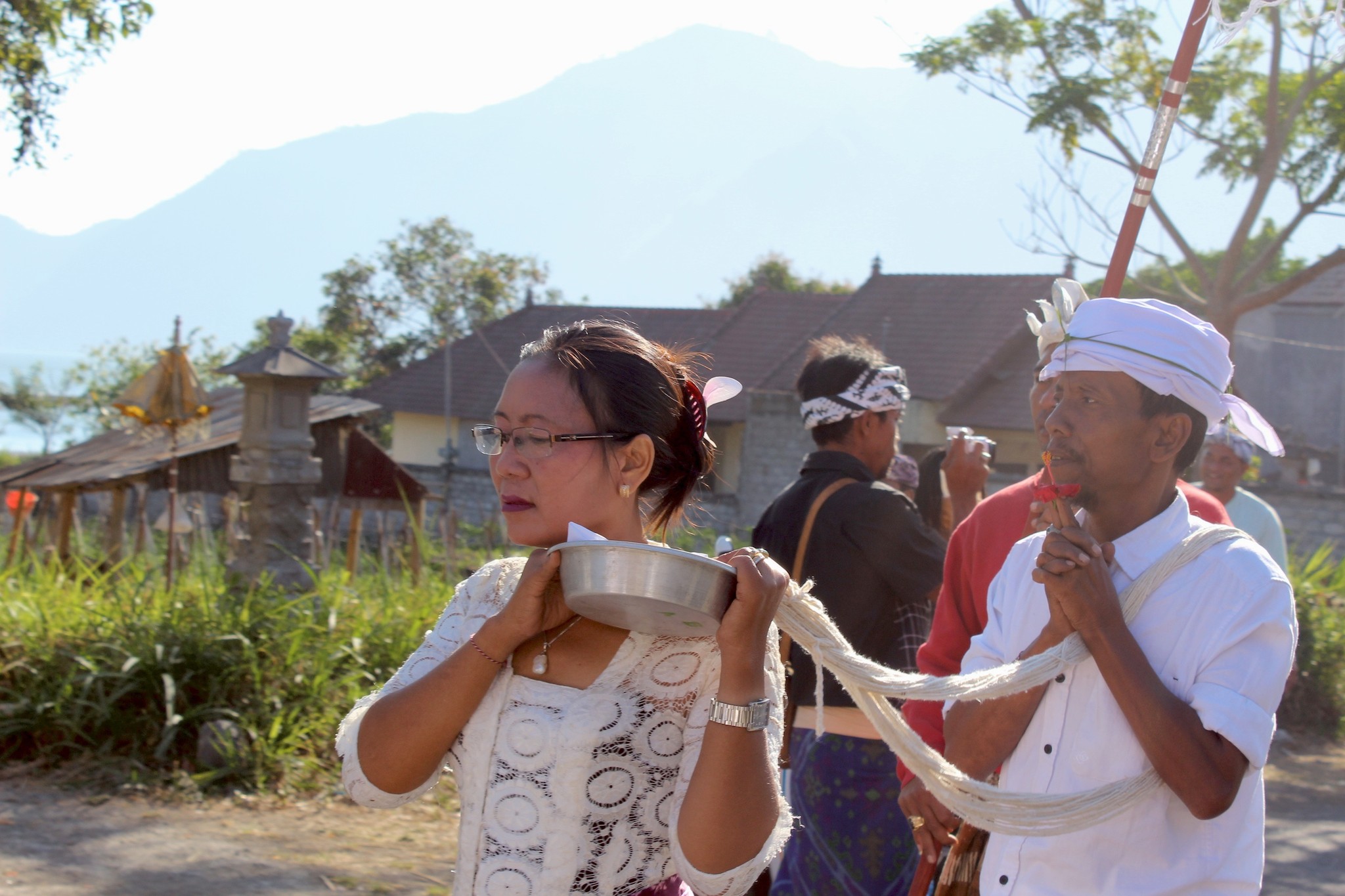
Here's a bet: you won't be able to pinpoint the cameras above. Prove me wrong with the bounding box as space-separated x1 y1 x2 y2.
946 435 996 465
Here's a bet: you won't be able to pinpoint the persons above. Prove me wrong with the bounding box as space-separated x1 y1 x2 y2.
939 296 1301 896
750 337 989 896
1186 417 1287 577
335 322 795 896
897 277 1232 896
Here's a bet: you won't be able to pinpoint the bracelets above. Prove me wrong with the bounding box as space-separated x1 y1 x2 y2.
469 634 506 669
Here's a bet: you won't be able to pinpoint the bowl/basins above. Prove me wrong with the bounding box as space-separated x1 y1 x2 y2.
547 540 737 637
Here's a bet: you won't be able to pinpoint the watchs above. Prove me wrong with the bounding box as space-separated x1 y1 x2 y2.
705 696 771 731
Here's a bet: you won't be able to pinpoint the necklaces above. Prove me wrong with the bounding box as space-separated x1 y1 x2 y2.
533 615 583 675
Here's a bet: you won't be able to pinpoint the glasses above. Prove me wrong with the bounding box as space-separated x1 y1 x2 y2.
470 423 639 460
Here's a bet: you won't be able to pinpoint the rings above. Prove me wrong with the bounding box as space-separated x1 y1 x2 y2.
750 550 765 565
1045 523 1062 535
757 548 769 558
907 815 924 831
